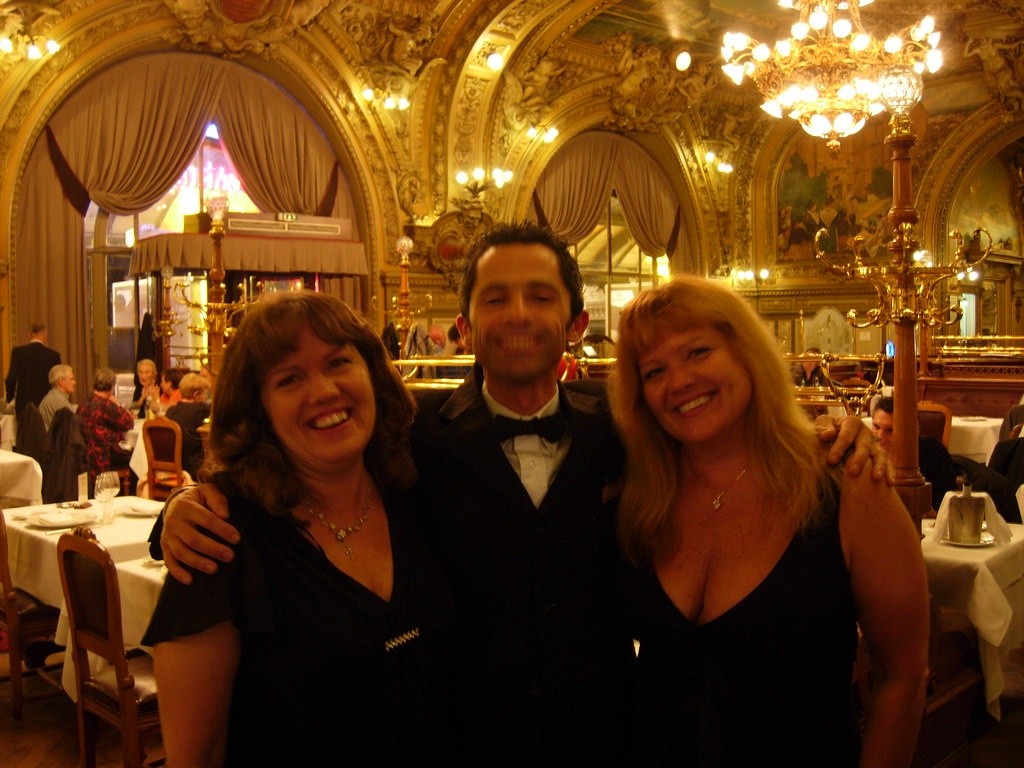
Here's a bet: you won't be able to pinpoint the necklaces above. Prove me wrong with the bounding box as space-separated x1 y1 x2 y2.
685 456 754 509
304 476 372 559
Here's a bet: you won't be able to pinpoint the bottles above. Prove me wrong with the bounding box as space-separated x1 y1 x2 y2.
886 338 894 357
963 481 972 497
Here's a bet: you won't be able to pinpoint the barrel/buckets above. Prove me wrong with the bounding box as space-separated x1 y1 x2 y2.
947 491 986 543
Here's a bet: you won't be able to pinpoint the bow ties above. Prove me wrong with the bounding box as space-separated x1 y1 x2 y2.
494 414 571 444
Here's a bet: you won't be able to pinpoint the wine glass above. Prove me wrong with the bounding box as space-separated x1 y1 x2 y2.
94 472 120 525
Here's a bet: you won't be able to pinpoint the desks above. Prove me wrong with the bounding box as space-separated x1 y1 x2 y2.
0 408 169 720
827 386 1024 723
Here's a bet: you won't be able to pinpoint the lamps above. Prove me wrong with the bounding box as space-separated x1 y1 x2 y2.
669 44 694 73
720 0 943 150
0 0 61 59
362 68 410 110
457 166 513 227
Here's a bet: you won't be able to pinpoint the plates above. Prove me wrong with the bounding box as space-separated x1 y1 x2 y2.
941 533 994 547
961 416 986 421
12 499 165 528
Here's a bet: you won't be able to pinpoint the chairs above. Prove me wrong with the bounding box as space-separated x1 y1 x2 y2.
18 402 54 504
143 415 182 502
917 400 952 449
840 377 874 417
47 406 130 501
1 508 61 722
57 525 166 768
851 591 984 768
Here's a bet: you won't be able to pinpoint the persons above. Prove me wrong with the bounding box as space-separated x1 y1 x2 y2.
140 221 457 768
5 323 61 427
130 358 218 483
73 369 139 497
150 218 900 768
863 395 959 517
611 277 927 767
38 365 77 432
